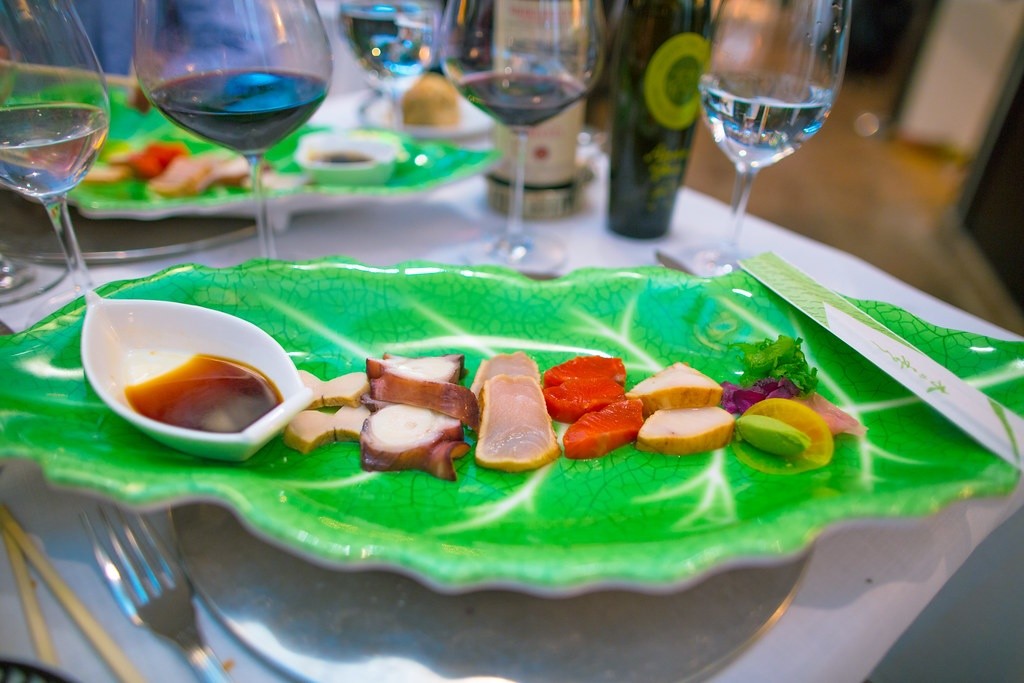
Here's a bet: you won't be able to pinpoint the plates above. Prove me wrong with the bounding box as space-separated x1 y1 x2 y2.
0 258 1023 599
175 502 808 683
0 57 498 222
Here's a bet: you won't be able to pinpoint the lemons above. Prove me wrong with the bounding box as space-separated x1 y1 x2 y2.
740 398 834 465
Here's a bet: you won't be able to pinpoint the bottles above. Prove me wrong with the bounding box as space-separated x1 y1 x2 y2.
605 1 713 237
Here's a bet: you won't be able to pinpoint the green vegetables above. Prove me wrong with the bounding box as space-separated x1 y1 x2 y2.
729 333 819 398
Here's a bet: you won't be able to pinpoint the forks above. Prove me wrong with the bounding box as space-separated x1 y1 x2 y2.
81 500 239 683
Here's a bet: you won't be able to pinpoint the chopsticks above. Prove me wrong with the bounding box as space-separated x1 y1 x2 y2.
0 506 149 683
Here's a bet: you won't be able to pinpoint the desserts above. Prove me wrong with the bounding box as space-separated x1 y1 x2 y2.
401 72 459 126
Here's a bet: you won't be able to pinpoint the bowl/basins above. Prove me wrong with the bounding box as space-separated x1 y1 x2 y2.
80 289 312 462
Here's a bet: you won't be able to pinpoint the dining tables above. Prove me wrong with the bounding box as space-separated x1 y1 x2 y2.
1 155 1024 681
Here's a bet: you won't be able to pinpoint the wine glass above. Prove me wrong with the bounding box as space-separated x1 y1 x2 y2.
664 0 855 282
438 2 607 277
0 0 112 332
130 0 335 258
340 1 440 131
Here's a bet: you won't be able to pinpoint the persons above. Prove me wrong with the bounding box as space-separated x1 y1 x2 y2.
1 0 276 86
